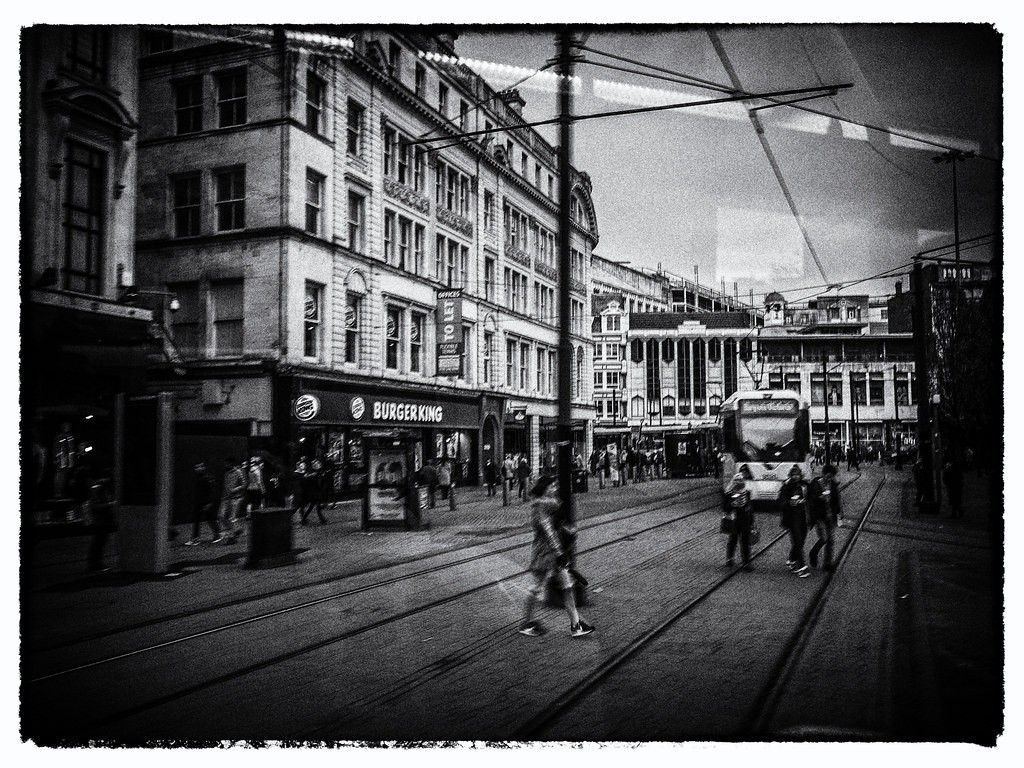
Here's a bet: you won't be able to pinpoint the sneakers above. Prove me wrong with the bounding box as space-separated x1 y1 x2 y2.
570 621 596 636
519 621 544 636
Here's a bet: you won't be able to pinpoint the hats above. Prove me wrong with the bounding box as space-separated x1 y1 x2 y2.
788 464 802 477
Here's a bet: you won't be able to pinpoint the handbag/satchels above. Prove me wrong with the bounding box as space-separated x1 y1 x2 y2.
749 527 760 545
719 515 735 534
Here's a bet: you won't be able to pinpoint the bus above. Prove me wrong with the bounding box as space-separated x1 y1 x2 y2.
721 388 813 503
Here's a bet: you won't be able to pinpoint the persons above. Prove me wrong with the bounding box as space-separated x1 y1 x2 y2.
776 466 811 578
30 444 52 523
912 446 973 528
374 458 387 492
388 458 403 500
816 440 885 471
807 464 845 572
519 475 596 637
76 441 95 482
577 445 720 488
422 453 531 510
722 482 755 572
53 423 75 468
183 457 336 546
87 467 119 576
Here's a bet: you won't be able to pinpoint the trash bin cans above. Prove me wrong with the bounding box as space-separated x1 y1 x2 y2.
244 505 295 569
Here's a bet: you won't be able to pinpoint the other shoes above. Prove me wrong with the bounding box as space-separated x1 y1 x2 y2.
808 553 818 568
185 536 202 545
785 559 797 568
822 563 836 573
743 564 754 571
211 534 223 543
726 560 736 566
790 566 811 578
